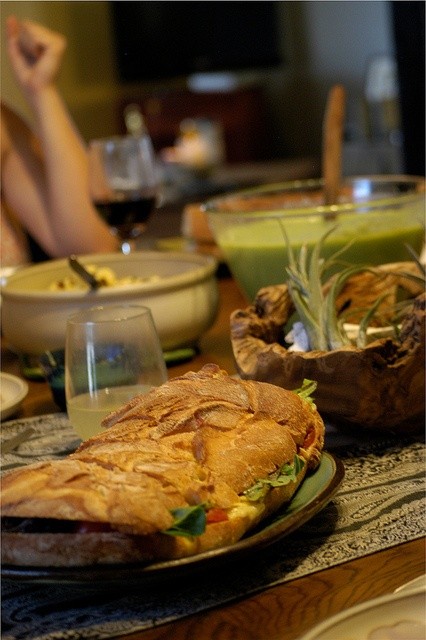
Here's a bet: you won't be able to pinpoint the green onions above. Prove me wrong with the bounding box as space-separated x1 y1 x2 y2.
270 209 425 350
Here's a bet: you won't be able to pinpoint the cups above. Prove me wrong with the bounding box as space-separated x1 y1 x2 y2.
63 304 170 446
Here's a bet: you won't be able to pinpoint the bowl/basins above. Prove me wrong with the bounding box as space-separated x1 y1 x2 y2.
0 251 218 356
200 172 425 308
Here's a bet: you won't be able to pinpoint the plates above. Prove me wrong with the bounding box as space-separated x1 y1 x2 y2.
0 410 345 577
0 371 30 416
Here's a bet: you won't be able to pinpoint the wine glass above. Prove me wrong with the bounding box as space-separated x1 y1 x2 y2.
86 136 157 256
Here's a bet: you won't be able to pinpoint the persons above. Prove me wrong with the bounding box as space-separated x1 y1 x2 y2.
0 14 122 266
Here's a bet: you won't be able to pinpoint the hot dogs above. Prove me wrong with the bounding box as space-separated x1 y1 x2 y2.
0 363 325 569
